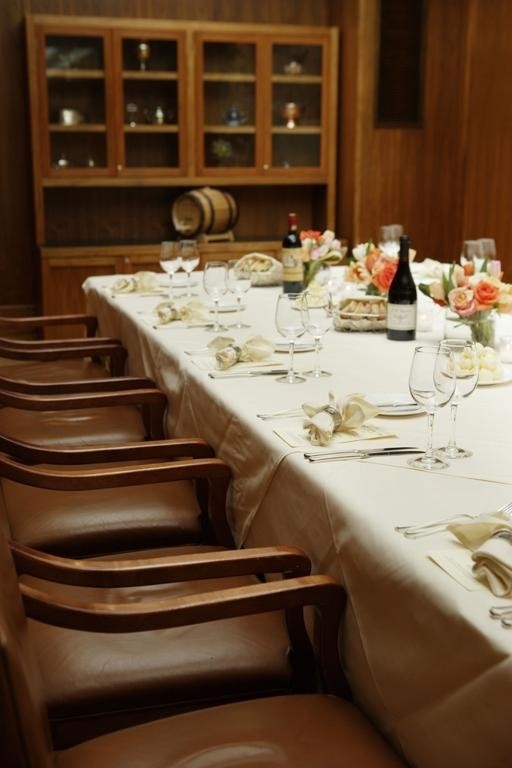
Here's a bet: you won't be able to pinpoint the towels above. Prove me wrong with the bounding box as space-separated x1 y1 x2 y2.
445 508 512 598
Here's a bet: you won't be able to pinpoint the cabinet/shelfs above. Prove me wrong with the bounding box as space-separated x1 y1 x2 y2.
25 13 339 339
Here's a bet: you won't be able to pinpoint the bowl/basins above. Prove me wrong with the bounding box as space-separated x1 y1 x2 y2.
52 110 89 124
321 304 387 333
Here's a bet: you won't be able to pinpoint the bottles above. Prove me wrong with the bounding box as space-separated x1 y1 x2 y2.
283 213 304 292
385 233 417 340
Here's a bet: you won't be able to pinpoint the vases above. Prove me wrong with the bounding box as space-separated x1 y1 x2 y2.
472 321 497 347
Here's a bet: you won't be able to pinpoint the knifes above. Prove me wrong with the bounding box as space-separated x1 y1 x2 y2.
304 444 424 461
211 368 294 380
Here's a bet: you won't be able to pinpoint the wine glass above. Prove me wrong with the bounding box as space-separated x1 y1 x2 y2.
406 345 456 471
227 260 251 329
301 289 332 379
433 337 478 459
205 260 230 334
179 239 201 299
273 293 309 384
158 240 181 300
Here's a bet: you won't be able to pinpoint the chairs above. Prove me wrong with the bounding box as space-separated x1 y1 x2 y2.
0 307 408 768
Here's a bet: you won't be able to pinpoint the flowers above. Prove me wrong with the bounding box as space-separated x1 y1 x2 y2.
418 256 504 347
298 229 348 287
343 237 400 299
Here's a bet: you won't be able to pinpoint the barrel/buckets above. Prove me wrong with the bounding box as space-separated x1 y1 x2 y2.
169 187 240 238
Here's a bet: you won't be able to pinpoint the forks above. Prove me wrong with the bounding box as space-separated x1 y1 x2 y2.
396 502 512 532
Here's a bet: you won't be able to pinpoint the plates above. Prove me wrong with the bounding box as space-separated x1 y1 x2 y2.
157 276 196 288
476 364 511 385
366 391 427 418
211 305 244 313
270 337 322 355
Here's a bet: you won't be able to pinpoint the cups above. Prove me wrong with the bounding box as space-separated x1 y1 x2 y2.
459 237 497 271
112 252 135 293
277 100 303 125
225 107 250 126
135 40 151 70
